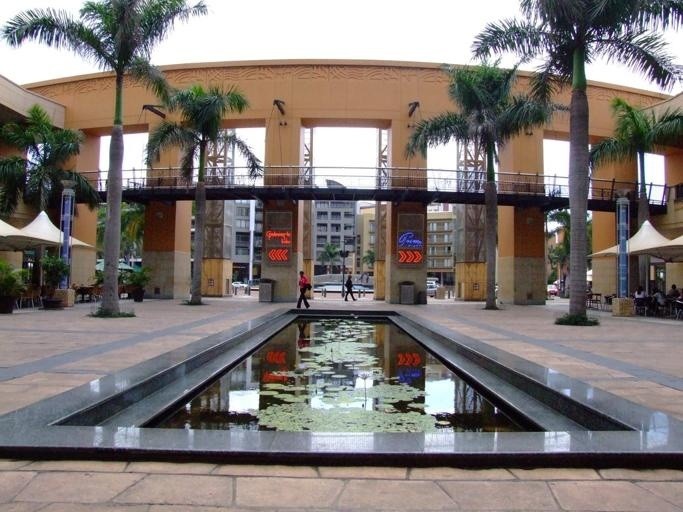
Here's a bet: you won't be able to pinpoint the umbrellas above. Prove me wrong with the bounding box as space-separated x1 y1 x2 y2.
0 210 95 287
586 220 683 297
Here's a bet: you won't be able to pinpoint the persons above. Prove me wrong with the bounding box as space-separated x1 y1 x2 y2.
297 271 310 308
669 284 683 299
635 285 644 298
345 275 357 301
651 288 668 306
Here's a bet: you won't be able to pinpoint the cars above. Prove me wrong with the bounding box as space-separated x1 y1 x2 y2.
232 281 248 288
546 284 560 297
426 278 440 297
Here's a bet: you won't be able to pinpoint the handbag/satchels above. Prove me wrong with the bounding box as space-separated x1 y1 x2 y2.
303 286 312 299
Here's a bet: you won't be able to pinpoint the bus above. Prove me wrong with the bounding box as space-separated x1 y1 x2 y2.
97 257 142 274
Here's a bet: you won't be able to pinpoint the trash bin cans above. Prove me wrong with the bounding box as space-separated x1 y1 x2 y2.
400 282 426 305
258 278 274 302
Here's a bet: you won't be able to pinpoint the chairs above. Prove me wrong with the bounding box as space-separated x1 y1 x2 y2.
15 282 42 310
586 290 683 320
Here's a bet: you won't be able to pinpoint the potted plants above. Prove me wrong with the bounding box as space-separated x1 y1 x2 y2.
0 259 31 314
38 253 71 309
127 265 152 302
